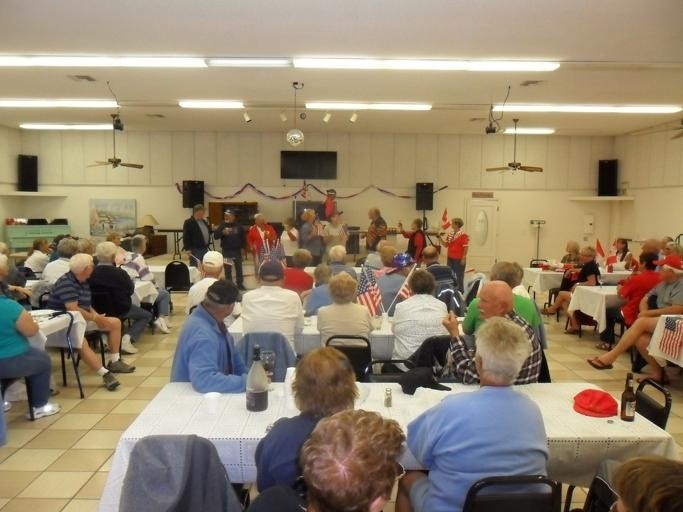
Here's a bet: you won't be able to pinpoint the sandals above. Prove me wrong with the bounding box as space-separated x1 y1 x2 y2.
596 343 612 350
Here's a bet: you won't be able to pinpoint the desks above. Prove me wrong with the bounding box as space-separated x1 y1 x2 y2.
150 235 167 255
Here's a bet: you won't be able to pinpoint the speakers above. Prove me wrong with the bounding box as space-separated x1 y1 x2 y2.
183 181 204 207
416 183 433 210
18 155 37 191
599 160 616 196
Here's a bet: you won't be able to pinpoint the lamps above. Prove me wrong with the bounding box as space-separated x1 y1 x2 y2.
286 82 305 147
242 112 252 124
350 113 358 123
143 215 158 233
322 113 333 123
280 112 287 123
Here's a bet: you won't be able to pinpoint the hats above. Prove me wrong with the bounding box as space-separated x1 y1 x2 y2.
194 205 205 210
224 209 234 215
573 389 618 417
203 251 223 267
260 260 284 281
653 256 682 270
208 280 242 303
385 252 415 274
398 367 451 395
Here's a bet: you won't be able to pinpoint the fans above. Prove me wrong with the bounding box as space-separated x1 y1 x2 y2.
86 114 144 169
486 118 543 174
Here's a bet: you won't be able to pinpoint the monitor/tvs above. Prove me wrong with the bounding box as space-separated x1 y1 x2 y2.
281 151 337 179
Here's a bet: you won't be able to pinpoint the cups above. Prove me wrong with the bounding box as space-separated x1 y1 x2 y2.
369 311 392 335
261 351 297 399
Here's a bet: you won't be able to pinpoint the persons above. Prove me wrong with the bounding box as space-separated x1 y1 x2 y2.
170 203 553 392
395 315 550 512
249 409 407 512
540 236 683 383
252 345 360 493
610 454 682 512
0 231 171 420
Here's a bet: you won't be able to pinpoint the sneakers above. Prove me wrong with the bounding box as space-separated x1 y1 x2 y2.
107 359 135 372
103 371 119 390
4 401 12 412
154 318 171 334
121 342 138 353
27 404 60 420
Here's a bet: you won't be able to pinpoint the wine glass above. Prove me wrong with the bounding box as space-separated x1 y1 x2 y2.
596 274 604 290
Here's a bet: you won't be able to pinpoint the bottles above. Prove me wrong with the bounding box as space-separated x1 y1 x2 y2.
245 343 268 411
621 372 636 422
607 263 613 272
384 388 391 407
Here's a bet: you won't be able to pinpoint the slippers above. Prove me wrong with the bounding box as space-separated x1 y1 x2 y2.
565 329 579 336
587 357 613 369
637 378 669 386
540 309 556 315
